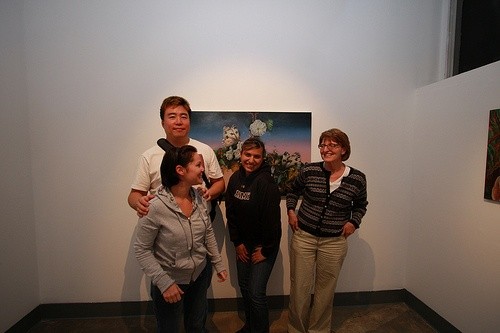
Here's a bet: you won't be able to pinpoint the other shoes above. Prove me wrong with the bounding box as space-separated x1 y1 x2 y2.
235 323 252 333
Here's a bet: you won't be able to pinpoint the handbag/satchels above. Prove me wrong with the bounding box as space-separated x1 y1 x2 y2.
209 194 223 223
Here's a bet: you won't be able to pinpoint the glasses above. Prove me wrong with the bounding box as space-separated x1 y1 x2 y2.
318 143 340 149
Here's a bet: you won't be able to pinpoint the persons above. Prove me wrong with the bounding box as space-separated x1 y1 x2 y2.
286 129 369 333
127 95 224 332
133 145 225 333
225 135 281 333
